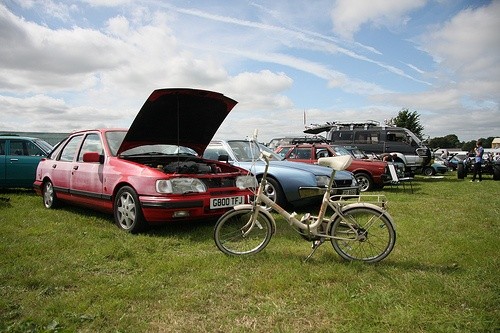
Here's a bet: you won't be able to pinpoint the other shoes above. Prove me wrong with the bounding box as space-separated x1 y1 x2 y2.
477 180 482 182
470 180 474 182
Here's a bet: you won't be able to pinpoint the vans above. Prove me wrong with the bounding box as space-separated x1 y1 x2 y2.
303 120 435 176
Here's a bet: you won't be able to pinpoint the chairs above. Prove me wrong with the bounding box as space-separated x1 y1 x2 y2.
387 162 413 194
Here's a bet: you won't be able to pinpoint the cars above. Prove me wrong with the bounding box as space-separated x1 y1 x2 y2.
186 139 360 214
0 136 55 189
33 88 261 235
266 134 405 193
422 147 500 181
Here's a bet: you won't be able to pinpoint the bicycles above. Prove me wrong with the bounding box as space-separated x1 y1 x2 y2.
213 129 396 265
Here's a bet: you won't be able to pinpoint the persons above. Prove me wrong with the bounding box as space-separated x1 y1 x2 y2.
468 141 484 182
441 151 446 159
382 154 397 163
485 152 500 162
